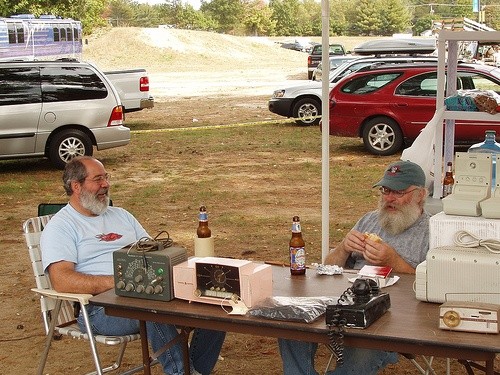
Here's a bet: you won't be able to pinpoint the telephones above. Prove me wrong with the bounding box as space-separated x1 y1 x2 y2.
326 278 391 329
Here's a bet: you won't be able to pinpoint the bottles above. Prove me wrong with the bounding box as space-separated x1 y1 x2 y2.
468 130 500 192
289 216 305 275
442 161 454 199
196 205 212 238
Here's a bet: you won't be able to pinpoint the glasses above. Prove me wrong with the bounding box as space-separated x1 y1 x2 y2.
379 186 418 197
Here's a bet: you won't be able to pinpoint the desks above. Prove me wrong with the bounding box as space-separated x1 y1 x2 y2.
88 258 500 375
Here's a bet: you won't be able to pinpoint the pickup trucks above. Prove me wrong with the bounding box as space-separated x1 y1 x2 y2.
307 44 348 82
104 69 154 113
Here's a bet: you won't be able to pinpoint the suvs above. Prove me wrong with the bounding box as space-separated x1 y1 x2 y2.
319 62 499 156
267 56 437 127
0 56 131 168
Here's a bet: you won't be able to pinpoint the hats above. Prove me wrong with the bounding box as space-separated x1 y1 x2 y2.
372 160 426 190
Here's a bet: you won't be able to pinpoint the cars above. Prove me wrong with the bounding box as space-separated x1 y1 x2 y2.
312 55 360 81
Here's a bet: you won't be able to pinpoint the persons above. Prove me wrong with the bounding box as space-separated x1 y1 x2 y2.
40 157 226 375
278 161 432 375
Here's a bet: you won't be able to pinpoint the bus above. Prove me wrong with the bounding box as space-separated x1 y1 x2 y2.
0 13 83 59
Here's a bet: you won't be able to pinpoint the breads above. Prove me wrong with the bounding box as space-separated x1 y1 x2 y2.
363 231 382 243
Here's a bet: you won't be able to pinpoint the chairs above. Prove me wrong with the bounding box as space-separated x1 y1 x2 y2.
37 198 114 341
334 48 340 52
22 213 162 375
